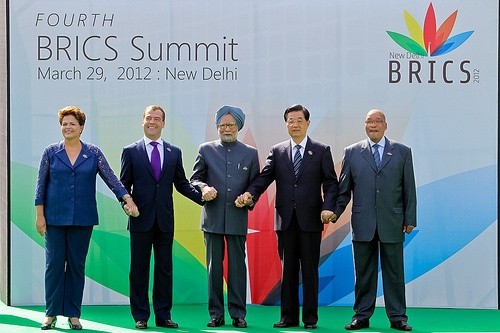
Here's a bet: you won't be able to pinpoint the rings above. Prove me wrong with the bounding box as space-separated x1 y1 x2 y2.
212 194 215 198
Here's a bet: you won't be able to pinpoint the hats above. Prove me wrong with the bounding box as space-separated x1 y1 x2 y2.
215 105 246 132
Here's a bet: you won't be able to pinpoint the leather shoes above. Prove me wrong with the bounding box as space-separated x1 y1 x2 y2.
207 318 222 327
304 325 317 329
391 320 412 331
136 320 147 329
156 319 178 328
233 318 247 328
345 319 369 330
274 321 288 327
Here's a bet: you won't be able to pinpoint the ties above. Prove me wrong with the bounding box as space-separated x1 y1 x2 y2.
149 142 161 181
293 145 303 178
372 143 380 170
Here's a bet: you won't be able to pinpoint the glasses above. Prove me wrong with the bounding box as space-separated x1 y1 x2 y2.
217 122 237 129
365 120 385 125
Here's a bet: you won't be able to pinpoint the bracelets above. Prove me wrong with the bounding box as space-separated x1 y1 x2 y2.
125 197 131 200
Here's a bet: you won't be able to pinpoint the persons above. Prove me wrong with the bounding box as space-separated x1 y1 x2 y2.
322 109 417 332
237 103 340 329
189 106 260 328
35 106 138 329
119 106 216 329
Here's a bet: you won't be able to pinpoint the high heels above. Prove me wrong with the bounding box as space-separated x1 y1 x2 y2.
68 318 82 330
41 316 57 330
